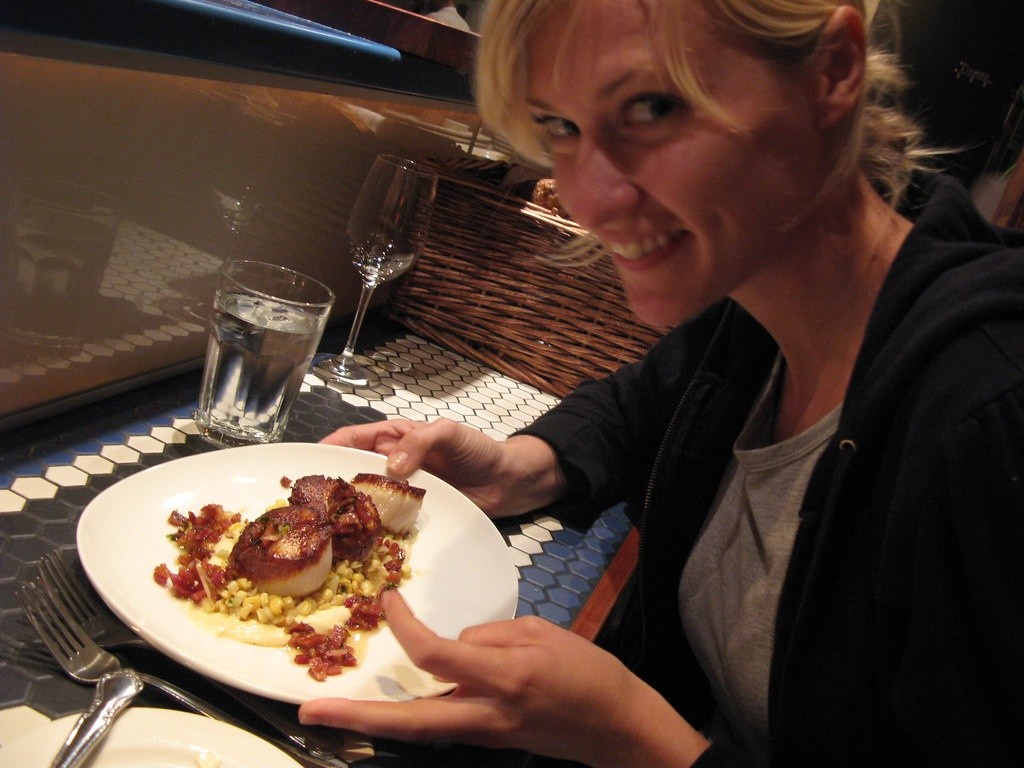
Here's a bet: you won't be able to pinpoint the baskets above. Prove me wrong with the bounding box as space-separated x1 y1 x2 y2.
379 149 669 401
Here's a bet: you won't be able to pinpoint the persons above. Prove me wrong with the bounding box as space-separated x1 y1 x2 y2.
298 0 1024 767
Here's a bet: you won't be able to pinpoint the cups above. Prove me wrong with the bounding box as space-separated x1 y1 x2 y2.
199 259 335 450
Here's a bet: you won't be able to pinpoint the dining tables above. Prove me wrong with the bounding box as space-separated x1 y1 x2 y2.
0 311 639 768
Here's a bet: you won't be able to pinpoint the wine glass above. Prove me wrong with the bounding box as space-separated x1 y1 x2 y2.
307 153 440 390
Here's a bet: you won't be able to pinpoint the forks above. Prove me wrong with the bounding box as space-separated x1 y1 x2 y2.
12 575 348 768
37 548 346 762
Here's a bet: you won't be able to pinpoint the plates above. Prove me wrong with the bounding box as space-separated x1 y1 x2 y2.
75 441 519 706
